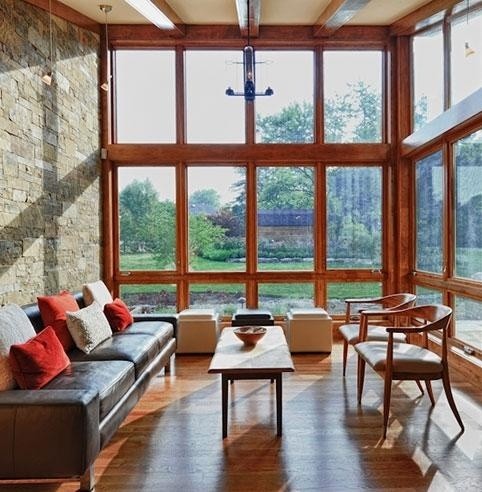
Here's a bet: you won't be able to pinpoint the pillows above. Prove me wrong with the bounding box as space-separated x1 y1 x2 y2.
36 289 82 352
80 278 113 313
64 299 114 355
1 299 42 391
9 323 73 391
103 297 134 334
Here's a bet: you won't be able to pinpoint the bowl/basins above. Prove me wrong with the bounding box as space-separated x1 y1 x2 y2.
232 326 268 345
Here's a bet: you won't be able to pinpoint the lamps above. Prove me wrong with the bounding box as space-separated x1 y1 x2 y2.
457 1 474 59
96 3 115 92
219 0 276 102
40 0 57 88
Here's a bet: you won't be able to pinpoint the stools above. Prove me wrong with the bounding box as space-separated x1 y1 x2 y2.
283 305 336 358
171 306 220 358
230 309 276 327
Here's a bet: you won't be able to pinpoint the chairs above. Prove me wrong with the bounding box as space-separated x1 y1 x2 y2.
338 291 418 404
355 302 465 443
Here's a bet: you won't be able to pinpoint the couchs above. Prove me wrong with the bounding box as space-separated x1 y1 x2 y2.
1 285 181 492
